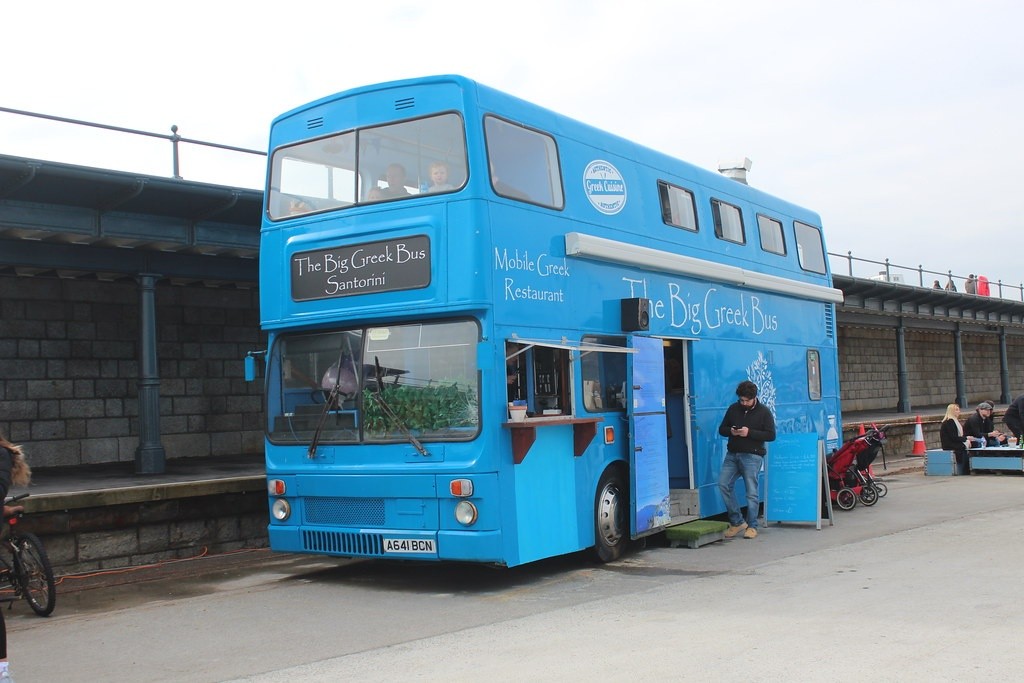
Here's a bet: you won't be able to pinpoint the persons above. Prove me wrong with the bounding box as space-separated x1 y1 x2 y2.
965 273 975 294
719 381 776 539
963 403 1000 448
976 274 990 296
380 162 411 198
367 186 384 200
933 280 943 290
1001 392 1024 445
0 434 34 683
940 404 978 475
945 280 957 292
428 160 454 193
507 364 521 402
982 400 1010 445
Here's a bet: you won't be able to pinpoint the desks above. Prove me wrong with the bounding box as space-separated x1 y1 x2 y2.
965 443 1024 474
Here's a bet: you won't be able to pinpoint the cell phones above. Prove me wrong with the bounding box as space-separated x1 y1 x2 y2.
733 426 740 430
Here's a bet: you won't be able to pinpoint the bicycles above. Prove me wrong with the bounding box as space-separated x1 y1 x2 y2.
0 492 55 616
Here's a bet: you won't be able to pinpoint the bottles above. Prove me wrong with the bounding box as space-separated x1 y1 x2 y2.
981 436 985 448
513 366 522 376
535 372 553 394
604 382 623 408
1019 435 1024 449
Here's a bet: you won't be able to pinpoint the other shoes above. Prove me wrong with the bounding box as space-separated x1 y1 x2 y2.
0 664 14 683
724 522 749 537
744 527 758 539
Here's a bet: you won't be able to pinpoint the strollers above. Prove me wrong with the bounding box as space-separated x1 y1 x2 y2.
825 424 891 510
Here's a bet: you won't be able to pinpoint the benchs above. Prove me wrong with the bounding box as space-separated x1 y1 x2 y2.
924 446 967 477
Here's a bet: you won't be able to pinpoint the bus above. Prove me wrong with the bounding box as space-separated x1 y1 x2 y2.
245 74 845 577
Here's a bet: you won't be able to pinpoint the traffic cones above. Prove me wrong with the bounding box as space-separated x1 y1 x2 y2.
857 424 872 476
905 416 926 457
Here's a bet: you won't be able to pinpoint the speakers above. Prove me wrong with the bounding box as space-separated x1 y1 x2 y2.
622 298 650 332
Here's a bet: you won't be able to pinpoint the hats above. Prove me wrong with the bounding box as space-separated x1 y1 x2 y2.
978 403 992 410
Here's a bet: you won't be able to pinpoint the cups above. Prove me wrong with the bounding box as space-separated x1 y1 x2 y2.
1008 440 1017 447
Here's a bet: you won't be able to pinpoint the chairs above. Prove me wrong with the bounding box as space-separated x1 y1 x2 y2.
312 367 360 429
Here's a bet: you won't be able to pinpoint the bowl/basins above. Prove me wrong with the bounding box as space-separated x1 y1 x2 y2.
508 406 526 420
